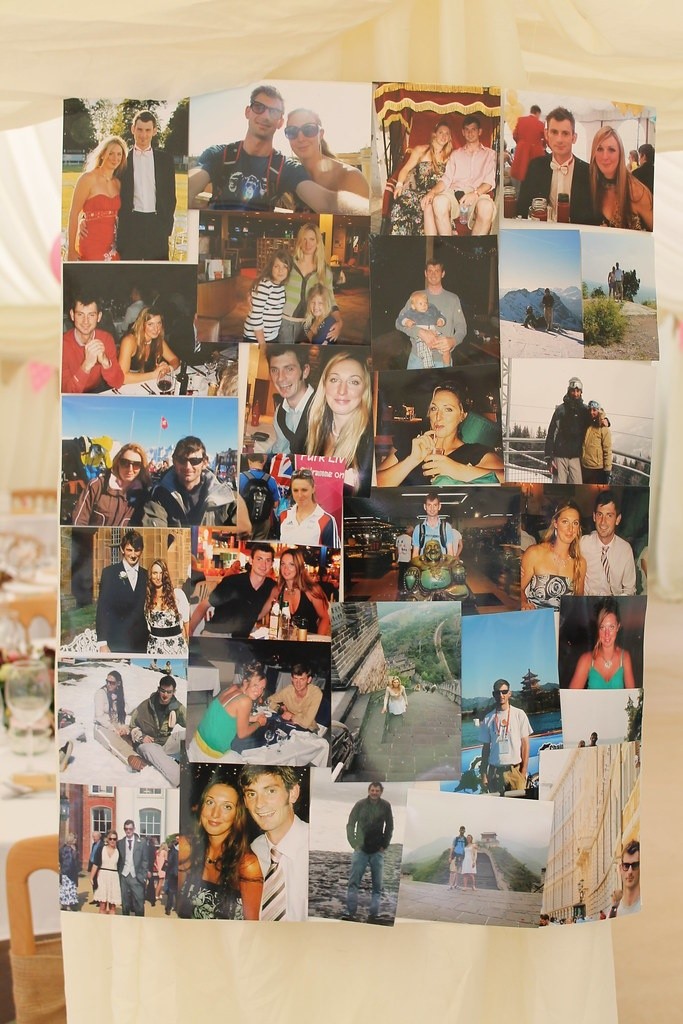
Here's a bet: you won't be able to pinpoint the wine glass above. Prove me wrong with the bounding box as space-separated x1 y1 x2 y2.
274 733 287 754
263 730 275 749
156 366 175 394
3 660 52 782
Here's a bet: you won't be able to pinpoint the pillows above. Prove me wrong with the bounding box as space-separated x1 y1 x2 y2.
458 409 503 452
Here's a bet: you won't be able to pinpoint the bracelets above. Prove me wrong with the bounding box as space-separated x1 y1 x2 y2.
474 190 480 197
396 182 403 187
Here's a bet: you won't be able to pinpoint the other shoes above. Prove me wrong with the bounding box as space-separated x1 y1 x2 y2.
368 916 374 923
341 914 352 920
447 883 478 891
89 900 100 907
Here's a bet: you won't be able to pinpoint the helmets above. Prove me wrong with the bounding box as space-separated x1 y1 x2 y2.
588 401 599 409
569 377 582 389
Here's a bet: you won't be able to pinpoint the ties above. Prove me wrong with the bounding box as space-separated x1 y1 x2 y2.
261 848 289 922
130 569 135 591
128 840 132 850
601 546 614 596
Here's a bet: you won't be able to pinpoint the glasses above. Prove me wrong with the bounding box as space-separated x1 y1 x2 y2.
105 679 116 686
283 123 320 139
175 454 203 466
622 862 639 871
124 828 133 831
251 100 283 121
159 687 174 694
119 458 142 470
109 838 116 841
292 470 312 477
496 690 508 694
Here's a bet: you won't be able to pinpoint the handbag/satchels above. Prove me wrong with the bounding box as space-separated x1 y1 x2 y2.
92 875 98 890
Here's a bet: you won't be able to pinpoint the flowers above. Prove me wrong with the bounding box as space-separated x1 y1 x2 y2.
119 570 129 585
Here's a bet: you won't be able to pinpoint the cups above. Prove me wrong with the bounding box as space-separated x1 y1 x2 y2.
427 448 445 480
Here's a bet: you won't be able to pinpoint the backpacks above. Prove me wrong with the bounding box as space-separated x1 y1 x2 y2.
242 470 274 524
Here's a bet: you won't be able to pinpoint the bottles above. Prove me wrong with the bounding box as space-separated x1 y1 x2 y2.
528 198 548 222
175 361 189 395
281 602 291 639
460 207 468 224
297 619 307 642
269 600 281 639
204 356 217 378
250 400 261 426
557 193 569 223
505 186 517 218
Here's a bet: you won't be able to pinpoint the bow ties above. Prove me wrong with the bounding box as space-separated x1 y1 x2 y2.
135 150 151 158
550 161 568 176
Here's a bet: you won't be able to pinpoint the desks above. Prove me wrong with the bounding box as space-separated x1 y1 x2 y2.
93 344 238 396
263 664 327 694
187 652 220 708
232 716 329 768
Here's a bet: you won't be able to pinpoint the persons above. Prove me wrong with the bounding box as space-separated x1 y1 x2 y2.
607 262 624 302
524 288 562 332
521 490 648 611
62 532 639 923
239 454 341 549
545 378 612 483
395 259 467 369
62 281 238 397
265 344 373 498
243 223 343 345
61 437 237 527
188 86 370 215
376 382 504 486
68 110 177 261
395 492 463 589
393 116 497 235
504 104 655 232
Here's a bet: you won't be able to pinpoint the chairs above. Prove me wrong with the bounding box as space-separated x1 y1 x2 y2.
190 580 218 604
